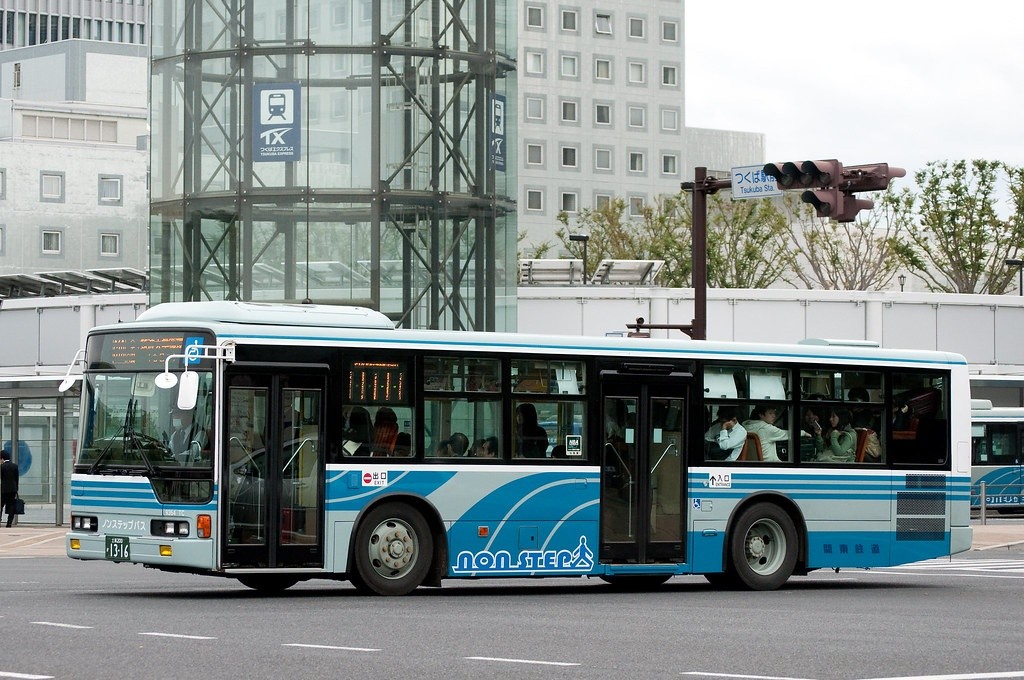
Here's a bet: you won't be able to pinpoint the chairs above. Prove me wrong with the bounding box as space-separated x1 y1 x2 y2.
736 433 765 461
346 403 570 458
854 429 869 462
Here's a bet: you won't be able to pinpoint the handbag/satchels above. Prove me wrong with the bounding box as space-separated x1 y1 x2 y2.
818 431 853 463
6 492 25 515
705 435 733 461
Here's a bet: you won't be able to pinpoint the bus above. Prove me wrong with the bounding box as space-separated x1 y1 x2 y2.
834 364 1024 519
56 301 975 599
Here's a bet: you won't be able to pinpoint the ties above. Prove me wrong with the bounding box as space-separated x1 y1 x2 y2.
180 431 185 445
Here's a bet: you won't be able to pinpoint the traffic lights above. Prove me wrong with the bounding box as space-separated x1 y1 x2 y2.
838 193 874 223
800 188 845 218
762 158 844 190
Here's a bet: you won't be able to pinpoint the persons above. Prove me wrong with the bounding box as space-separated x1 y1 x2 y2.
602 397 685 509
467 437 498 457
0 450 19 527
262 390 302 544
705 386 910 461
392 432 411 456
515 403 549 457
551 445 566 458
168 414 209 462
434 432 469 457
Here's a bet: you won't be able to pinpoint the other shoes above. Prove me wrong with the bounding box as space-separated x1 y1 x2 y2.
6 524 11 528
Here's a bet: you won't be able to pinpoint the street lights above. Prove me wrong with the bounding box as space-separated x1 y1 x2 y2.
569 234 591 286
1005 260 1024 296
897 274 906 292
